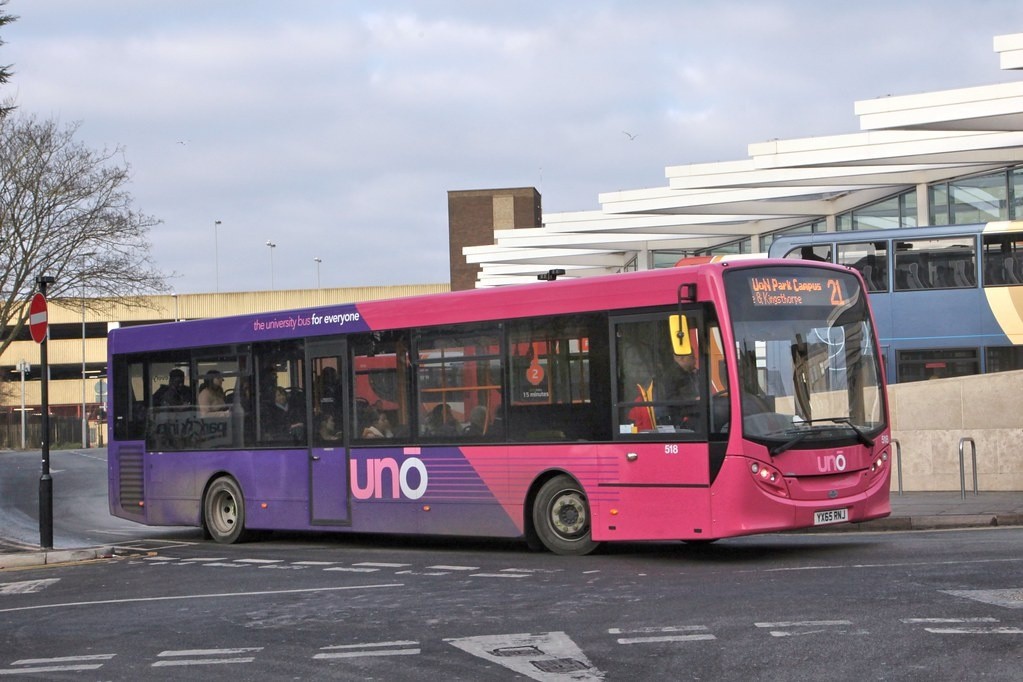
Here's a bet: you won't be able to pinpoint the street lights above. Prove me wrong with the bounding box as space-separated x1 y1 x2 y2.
266 240 276 290
214 219 222 293
77 250 96 447
314 258 322 289
171 293 178 323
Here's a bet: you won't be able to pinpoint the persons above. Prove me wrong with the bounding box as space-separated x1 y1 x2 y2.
152 369 192 405
429 403 463 438
260 366 281 400
263 386 305 443
312 413 342 440
360 408 395 438
467 406 492 439
313 366 344 419
198 370 234 418
657 346 704 431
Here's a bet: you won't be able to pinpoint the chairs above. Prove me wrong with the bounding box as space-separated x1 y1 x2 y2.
858 253 1023 292
645 351 671 425
283 387 303 400
223 389 233 409
355 397 369 408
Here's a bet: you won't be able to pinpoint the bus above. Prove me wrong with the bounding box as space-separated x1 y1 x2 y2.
107 257 892 555
677 220 1023 396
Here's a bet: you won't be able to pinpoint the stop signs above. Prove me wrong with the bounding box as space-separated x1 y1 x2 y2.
28 293 49 346
